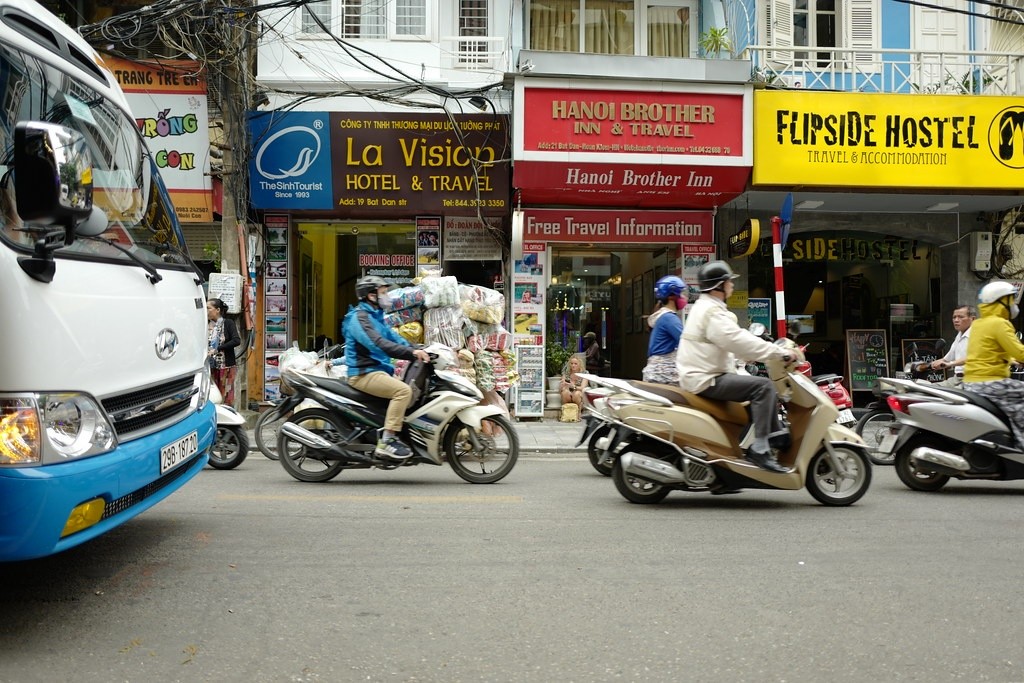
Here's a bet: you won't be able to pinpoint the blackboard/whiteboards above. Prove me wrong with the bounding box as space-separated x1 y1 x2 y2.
844 329 889 391
901 339 946 383
747 298 771 335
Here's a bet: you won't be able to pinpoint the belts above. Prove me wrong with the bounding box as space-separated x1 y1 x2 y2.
957 373 962 377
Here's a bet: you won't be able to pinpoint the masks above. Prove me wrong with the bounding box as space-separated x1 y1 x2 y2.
1009 305 1019 320
378 294 394 309
674 296 687 310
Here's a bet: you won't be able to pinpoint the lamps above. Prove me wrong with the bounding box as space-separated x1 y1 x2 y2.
469 96 487 111
747 72 766 83
520 58 535 75
252 90 268 106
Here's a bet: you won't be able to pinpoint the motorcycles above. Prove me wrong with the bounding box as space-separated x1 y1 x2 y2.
201 375 249 470
255 345 520 483
854 335 959 465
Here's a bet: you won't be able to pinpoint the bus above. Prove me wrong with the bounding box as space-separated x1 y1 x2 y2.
0 0 217 565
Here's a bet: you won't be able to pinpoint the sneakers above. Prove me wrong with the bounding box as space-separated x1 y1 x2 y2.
374 437 414 459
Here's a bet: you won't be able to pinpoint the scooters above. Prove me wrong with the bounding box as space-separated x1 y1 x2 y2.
756 344 857 432
574 322 874 506
875 331 1024 492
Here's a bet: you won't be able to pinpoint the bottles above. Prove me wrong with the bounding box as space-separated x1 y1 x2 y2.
519 348 542 388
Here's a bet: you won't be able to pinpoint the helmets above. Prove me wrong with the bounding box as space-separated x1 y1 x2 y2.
355 275 393 301
655 275 687 302
698 260 740 292
978 281 1018 304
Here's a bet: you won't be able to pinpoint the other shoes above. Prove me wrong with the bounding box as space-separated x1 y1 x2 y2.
745 445 796 473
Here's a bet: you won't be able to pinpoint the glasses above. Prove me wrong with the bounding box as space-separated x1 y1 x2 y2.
1012 294 1018 299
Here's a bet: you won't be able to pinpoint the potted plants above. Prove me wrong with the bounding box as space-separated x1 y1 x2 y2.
545 328 576 391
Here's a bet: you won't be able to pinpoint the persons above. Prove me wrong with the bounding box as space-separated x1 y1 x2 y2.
676 261 798 474
583 332 599 375
342 276 430 459
642 275 688 388
927 304 976 390
325 355 345 370
559 355 589 422
207 298 241 407
962 281 1024 452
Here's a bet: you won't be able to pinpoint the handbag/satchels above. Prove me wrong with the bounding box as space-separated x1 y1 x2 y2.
559 402 577 422
